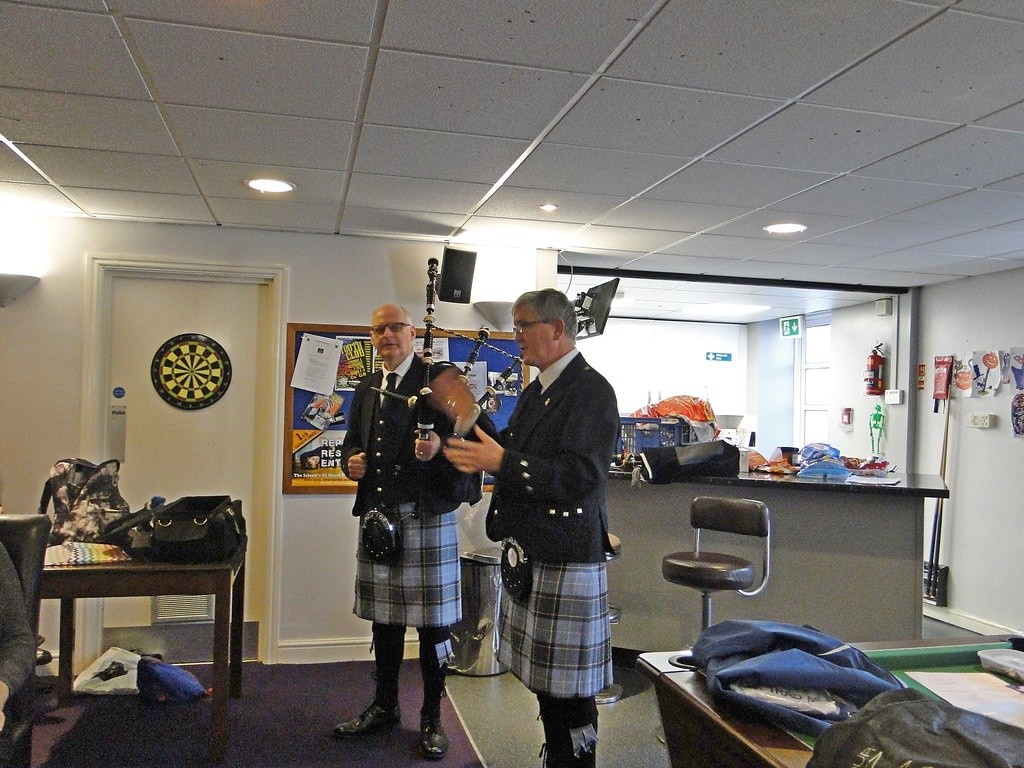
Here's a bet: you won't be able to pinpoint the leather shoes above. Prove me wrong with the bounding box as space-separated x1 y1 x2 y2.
333 702 400 738
420 709 448 758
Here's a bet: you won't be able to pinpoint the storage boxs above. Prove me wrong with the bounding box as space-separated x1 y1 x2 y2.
976 649 1024 684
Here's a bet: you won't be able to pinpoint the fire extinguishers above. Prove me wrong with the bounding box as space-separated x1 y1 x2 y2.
863 342 886 395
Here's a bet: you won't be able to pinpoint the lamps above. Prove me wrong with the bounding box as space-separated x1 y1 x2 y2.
475 300 517 330
0 275 40 310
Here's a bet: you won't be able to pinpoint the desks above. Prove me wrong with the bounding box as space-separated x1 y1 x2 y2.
35 540 254 765
639 620 1024 768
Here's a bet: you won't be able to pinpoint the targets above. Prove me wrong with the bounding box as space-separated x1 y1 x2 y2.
150 332 232 411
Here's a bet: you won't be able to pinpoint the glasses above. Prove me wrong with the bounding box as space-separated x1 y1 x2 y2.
370 323 411 335
512 318 552 334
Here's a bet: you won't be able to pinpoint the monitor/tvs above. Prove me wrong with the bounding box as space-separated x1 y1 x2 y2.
576 278 620 339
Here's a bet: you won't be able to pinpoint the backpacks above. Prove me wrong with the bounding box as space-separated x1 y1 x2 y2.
39 458 130 544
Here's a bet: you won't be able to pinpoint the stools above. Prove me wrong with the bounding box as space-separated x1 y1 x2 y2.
654 495 775 744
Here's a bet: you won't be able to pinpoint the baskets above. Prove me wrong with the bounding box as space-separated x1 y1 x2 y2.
615 416 715 464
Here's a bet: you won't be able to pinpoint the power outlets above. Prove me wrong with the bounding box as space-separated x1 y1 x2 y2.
968 414 996 428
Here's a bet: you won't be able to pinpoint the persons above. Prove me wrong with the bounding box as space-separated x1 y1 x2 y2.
0 544 38 768
340 304 494 758
444 290 620 768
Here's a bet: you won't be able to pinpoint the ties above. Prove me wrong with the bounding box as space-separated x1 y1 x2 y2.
381 373 398 409
525 380 543 413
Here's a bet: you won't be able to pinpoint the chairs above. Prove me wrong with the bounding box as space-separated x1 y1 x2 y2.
0 509 58 765
590 527 623 705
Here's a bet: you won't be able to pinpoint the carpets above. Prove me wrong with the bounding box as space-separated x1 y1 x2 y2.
20 652 489 766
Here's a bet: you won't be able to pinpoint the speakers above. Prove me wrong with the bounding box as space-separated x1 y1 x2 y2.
436 246 478 303
876 300 891 316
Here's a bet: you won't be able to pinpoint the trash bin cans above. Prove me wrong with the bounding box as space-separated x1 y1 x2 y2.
450 552 509 676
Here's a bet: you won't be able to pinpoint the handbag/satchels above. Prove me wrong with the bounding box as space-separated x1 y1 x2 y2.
95 495 246 562
361 507 403 565
500 537 532 602
